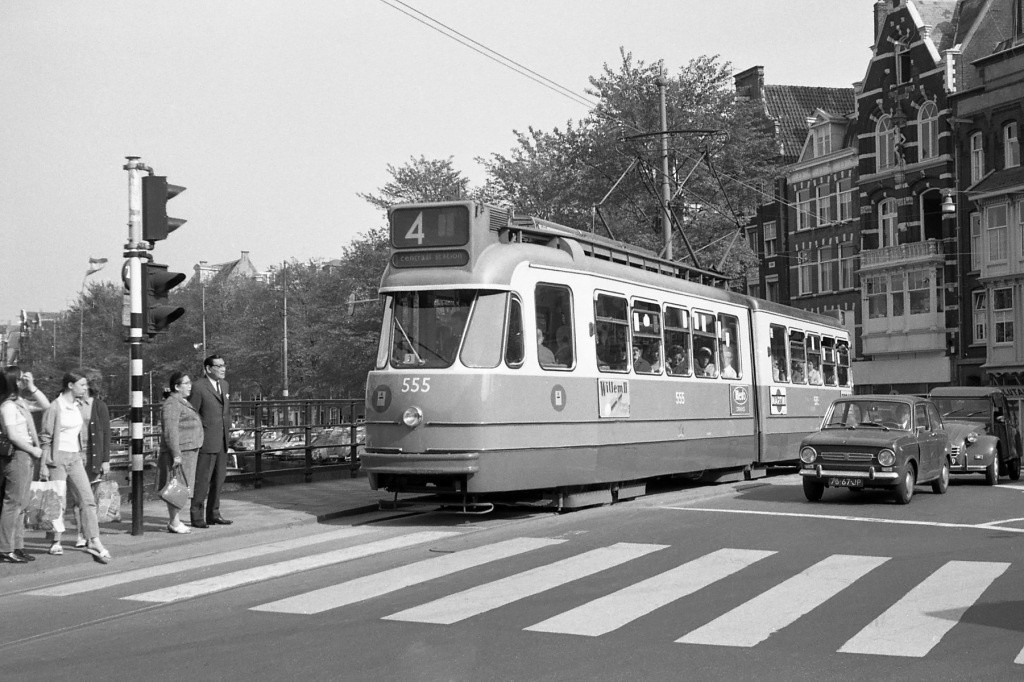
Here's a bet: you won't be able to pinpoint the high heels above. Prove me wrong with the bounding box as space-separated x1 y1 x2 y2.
0 552 27 563
14 549 35 561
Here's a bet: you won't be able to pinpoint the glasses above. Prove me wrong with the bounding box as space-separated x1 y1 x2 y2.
211 364 226 367
178 381 192 384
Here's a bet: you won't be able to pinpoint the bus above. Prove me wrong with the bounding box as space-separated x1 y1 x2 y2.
347 129 860 517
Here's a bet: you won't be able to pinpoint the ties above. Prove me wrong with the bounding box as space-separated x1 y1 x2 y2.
900 424 904 429
216 381 220 395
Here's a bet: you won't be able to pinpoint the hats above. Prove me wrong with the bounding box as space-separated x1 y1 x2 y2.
699 346 711 356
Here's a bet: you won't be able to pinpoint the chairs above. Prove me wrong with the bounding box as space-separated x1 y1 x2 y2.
555 327 572 365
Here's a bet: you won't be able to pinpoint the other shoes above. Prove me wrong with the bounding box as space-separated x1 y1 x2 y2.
75 541 85 547
168 521 192 533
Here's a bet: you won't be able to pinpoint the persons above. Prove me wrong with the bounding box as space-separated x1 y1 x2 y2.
0 366 112 563
435 312 740 379
772 357 821 385
885 404 910 429
158 371 205 533
187 355 234 529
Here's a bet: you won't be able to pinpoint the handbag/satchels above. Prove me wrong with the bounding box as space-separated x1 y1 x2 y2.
91 471 121 523
25 478 66 533
158 463 190 510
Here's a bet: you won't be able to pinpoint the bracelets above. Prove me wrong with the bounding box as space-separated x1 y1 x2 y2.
30 387 38 394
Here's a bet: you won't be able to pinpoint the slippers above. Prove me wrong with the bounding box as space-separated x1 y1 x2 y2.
86 549 111 562
50 545 63 555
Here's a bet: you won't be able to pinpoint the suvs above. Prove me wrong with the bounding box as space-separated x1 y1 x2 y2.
797 393 953 506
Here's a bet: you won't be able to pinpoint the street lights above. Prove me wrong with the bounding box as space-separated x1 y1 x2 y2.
78 264 105 370
200 267 221 361
282 259 294 399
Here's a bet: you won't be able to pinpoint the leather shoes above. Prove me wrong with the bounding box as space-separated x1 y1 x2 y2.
207 517 233 525
191 523 209 528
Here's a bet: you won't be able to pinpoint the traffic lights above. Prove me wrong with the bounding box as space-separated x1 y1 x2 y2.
140 261 186 340
141 174 189 242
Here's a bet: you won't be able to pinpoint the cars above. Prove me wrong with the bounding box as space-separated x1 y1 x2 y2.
927 385 1022 485
144 417 367 467
